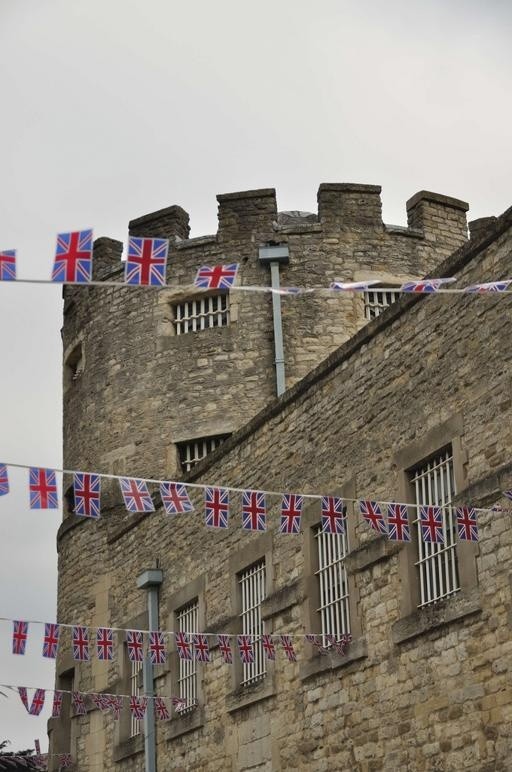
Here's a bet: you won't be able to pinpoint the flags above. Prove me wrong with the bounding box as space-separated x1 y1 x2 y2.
358 499 388 534
119 478 156 512
387 503 411 542
11 620 28 655
176 631 353 663
96 628 113 660
463 279 511 294
148 632 167 664
205 487 229 529
333 279 383 292
400 278 455 293
192 262 241 288
52 228 94 282
17 687 186 721
160 483 195 514
73 627 89 662
30 466 58 509
73 472 100 518
0 751 73 771
281 494 302 534
126 631 144 662
1 464 9 496
259 284 315 297
242 491 266 532
42 623 59 658
456 506 479 542
321 495 348 535
420 507 444 543
1 249 17 280
124 237 170 285
